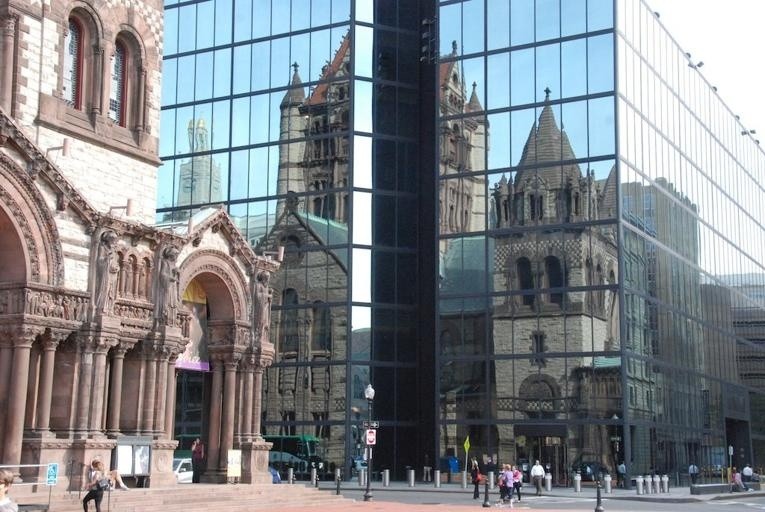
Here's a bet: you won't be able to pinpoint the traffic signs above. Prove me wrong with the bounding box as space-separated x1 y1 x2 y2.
363 421 380 428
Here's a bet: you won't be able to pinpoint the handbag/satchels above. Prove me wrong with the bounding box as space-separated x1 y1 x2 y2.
498 479 502 486
96 478 108 490
476 472 480 480
512 481 521 489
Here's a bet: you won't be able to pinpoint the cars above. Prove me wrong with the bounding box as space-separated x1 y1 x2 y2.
351 461 368 476
269 465 281 484
518 459 530 484
173 458 193 483
575 461 608 480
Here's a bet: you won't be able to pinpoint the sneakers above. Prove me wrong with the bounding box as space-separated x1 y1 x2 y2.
119 485 129 491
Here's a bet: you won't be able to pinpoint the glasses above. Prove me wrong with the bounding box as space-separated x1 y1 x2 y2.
0 481 5 485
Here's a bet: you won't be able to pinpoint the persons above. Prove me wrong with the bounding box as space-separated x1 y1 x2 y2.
688 462 699 485
616 458 626 486
190 437 205 483
0 468 18 512
105 468 128 491
732 463 759 491
422 453 433 482
468 456 546 505
82 462 107 512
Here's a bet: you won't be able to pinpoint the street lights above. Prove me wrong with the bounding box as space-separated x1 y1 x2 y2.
611 436 621 441
363 384 375 503
611 413 621 485
729 445 734 493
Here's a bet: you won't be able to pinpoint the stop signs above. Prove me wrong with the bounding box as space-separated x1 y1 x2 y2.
366 429 376 446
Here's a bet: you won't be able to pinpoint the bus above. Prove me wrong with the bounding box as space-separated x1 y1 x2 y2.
173 435 321 479
700 446 725 478
